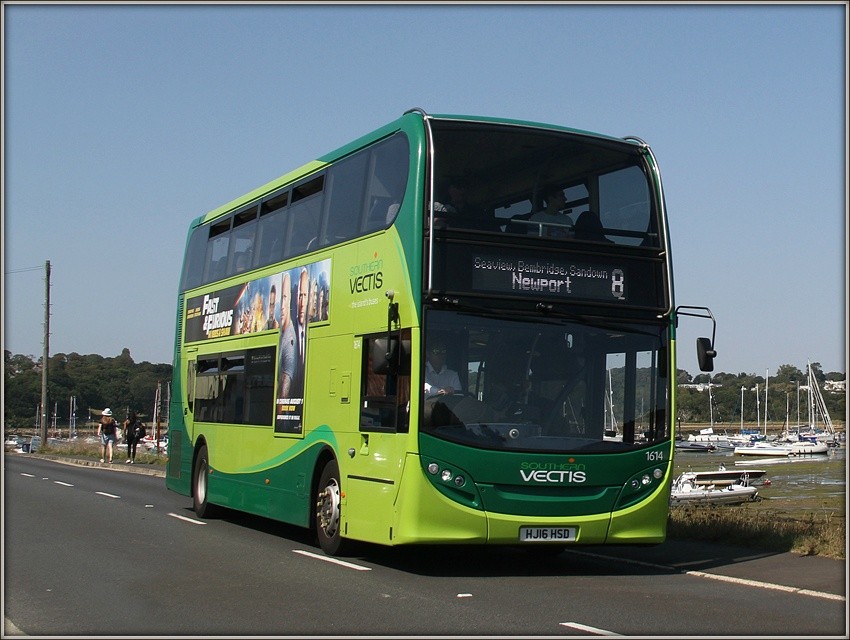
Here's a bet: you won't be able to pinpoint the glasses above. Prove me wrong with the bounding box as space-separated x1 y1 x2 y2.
554 192 565 199
432 346 449 354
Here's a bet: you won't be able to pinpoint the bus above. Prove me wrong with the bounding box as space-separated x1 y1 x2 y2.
165 106 717 555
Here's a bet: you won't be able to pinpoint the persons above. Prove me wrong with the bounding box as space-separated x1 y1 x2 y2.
122 410 147 466
260 285 280 331
528 186 575 239
309 271 328 323
96 408 119 466
407 338 464 421
489 348 594 448
293 268 309 412
387 185 447 229
278 272 297 423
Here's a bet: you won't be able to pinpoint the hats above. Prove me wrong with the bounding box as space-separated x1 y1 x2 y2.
102 408 113 416
127 409 135 414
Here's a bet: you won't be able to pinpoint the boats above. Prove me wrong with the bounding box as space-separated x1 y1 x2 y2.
671 477 760 506
678 464 767 485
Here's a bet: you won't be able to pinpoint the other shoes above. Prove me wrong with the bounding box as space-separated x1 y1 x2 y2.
100 459 104 463
125 459 135 464
109 460 114 463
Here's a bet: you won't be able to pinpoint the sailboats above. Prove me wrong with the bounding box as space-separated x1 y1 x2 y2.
48 379 168 456
673 358 846 456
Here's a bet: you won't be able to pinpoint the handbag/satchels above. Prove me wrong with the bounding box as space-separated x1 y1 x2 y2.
97 424 103 436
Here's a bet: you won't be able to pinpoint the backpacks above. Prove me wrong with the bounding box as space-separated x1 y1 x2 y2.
103 422 114 436
132 420 145 438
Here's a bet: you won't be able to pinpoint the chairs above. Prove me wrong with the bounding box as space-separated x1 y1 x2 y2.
204 200 528 284
